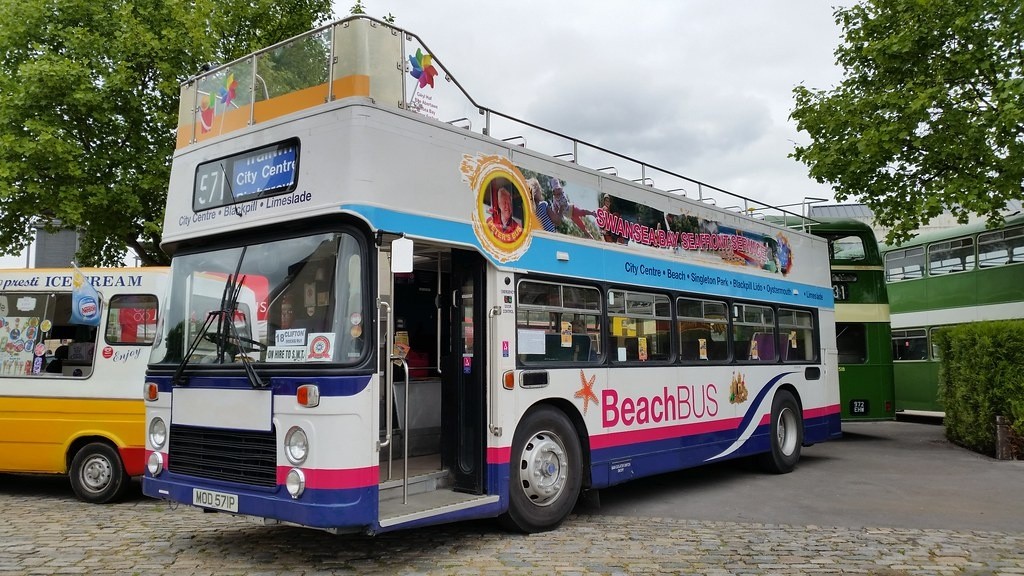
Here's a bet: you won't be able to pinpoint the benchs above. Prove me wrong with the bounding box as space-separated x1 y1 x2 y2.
521 332 789 361
446 118 766 221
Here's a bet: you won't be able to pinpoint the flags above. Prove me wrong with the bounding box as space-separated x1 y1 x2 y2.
68 268 102 328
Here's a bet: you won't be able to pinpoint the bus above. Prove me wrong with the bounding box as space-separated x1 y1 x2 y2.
838 212 1024 413
139 12 844 539
655 215 897 424
0 268 270 503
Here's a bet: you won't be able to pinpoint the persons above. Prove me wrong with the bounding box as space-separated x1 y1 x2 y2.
569 319 598 361
492 188 518 234
